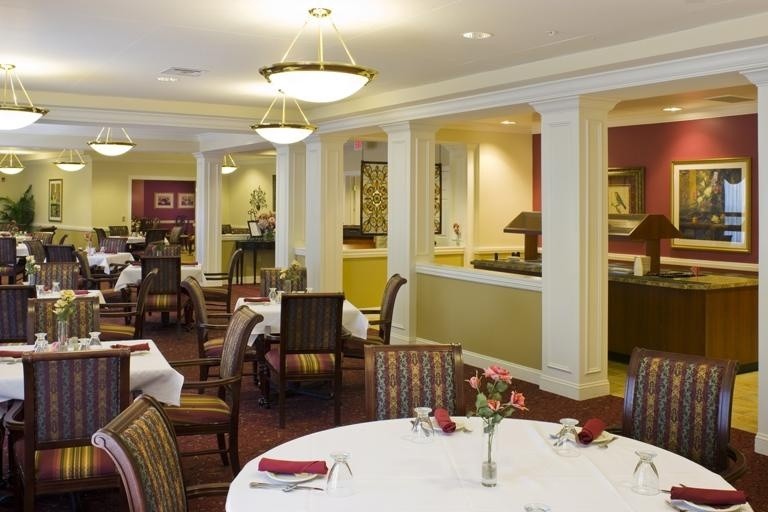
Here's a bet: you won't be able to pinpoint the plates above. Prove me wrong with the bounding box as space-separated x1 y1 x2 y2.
666 484 742 511
565 427 613 443
265 470 317 483
421 417 464 432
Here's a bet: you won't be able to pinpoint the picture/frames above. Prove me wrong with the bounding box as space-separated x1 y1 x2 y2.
48 178 64 222
669 156 753 253
154 191 175 210
607 164 647 244
176 191 195 210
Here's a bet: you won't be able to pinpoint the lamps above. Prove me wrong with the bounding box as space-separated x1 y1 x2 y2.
0 152 26 176
222 152 241 176
86 124 138 158
51 146 87 172
0 62 51 132
258 6 379 105
249 89 321 145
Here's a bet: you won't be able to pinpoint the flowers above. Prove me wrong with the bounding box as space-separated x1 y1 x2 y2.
247 184 269 221
465 365 529 478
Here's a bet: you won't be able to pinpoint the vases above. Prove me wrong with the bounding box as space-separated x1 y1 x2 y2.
482 461 498 488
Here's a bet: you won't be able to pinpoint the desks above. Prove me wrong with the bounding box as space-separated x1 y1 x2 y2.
224 415 754 511
235 295 371 409
609 276 753 375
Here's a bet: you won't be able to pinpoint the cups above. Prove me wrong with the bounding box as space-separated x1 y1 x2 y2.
77 246 106 257
129 231 147 238
36 281 62 298
14 230 27 237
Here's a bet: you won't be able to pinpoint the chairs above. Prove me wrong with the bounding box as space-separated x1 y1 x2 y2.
262 292 345 428
0 213 308 276
334 276 407 388
363 344 466 423
604 346 746 480
1 276 264 511
260 267 309 296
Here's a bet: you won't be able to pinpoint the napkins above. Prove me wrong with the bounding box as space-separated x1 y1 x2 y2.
435 408 456 434
243 298 270 303
577 418 605 445
257 457 328 475
670 487 747 508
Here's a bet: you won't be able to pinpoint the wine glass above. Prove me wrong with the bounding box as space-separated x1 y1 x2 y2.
31 332 103 353
555 417 581 457
326 450 356 497
630 448 662 496
268 286 313 304
412 406 434 444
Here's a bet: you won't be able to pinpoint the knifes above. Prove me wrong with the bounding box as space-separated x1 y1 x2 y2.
248 482 323 491
